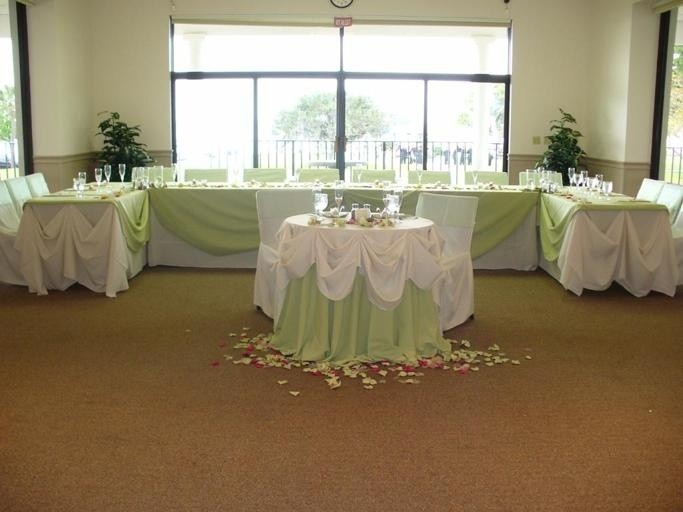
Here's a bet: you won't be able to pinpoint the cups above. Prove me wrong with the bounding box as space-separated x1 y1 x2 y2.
73 163 177 199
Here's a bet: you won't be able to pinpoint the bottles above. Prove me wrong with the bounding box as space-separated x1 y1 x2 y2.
351 203 371 220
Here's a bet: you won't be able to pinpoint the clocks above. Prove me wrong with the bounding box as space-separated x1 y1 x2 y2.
330 0 353 8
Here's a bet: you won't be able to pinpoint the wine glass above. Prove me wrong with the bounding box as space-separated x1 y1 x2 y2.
295 168 301 185
416 164 424 185
472 169 479 185
355 164 363 184
311 180 344 219
525 166 613 202
383 184 403 226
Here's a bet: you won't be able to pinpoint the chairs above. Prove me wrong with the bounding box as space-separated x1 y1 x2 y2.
252 188 315 320
353 168 396 179
242 168 287 180
413 191 478 331
295 168 340 180
518 170 564 186
184 167 229 181
633 178 683 286
131 166 178 180
464 170 510 185
0 172 51 287
408 170 451 186
